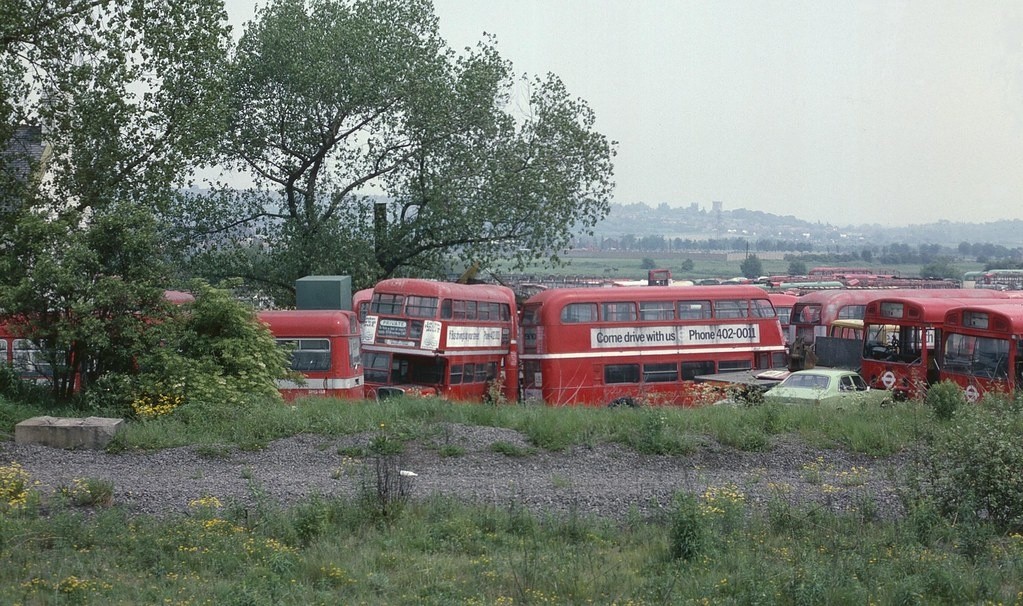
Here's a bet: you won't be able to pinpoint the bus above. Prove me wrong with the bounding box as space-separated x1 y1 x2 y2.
0 288 365 404
519 283 784 412
652 265 1023 404
363 279 519 408
350 286 625 384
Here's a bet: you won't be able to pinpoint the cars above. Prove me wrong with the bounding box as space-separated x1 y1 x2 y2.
760 369 893 409
